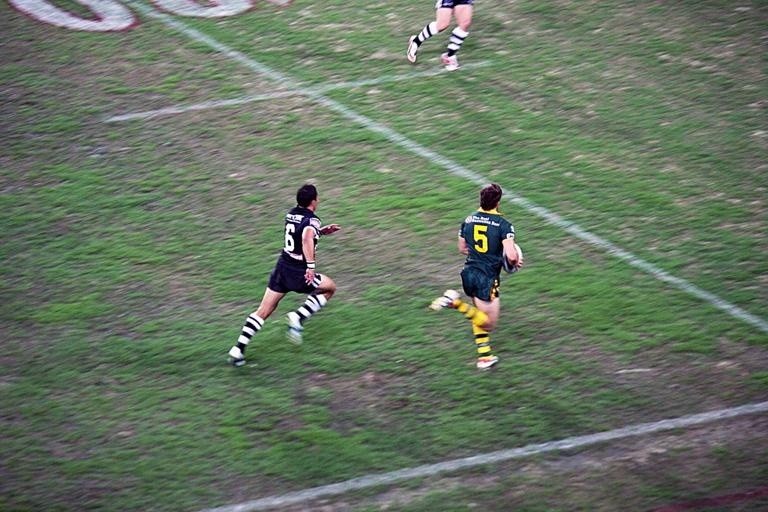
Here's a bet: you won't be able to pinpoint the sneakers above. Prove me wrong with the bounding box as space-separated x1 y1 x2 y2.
477 356 498 368
286 311 303 346
228 345 246 367
407 34 419 63
430 288 458 311
441 51 458 72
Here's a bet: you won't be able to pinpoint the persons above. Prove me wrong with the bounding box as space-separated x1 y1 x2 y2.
428 181 522 370
227 184 342 368
406 0 474 70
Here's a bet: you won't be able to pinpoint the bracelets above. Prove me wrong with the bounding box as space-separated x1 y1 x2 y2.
306 261 316 270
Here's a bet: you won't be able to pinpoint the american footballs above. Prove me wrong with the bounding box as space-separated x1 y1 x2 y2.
503 244 523 273
